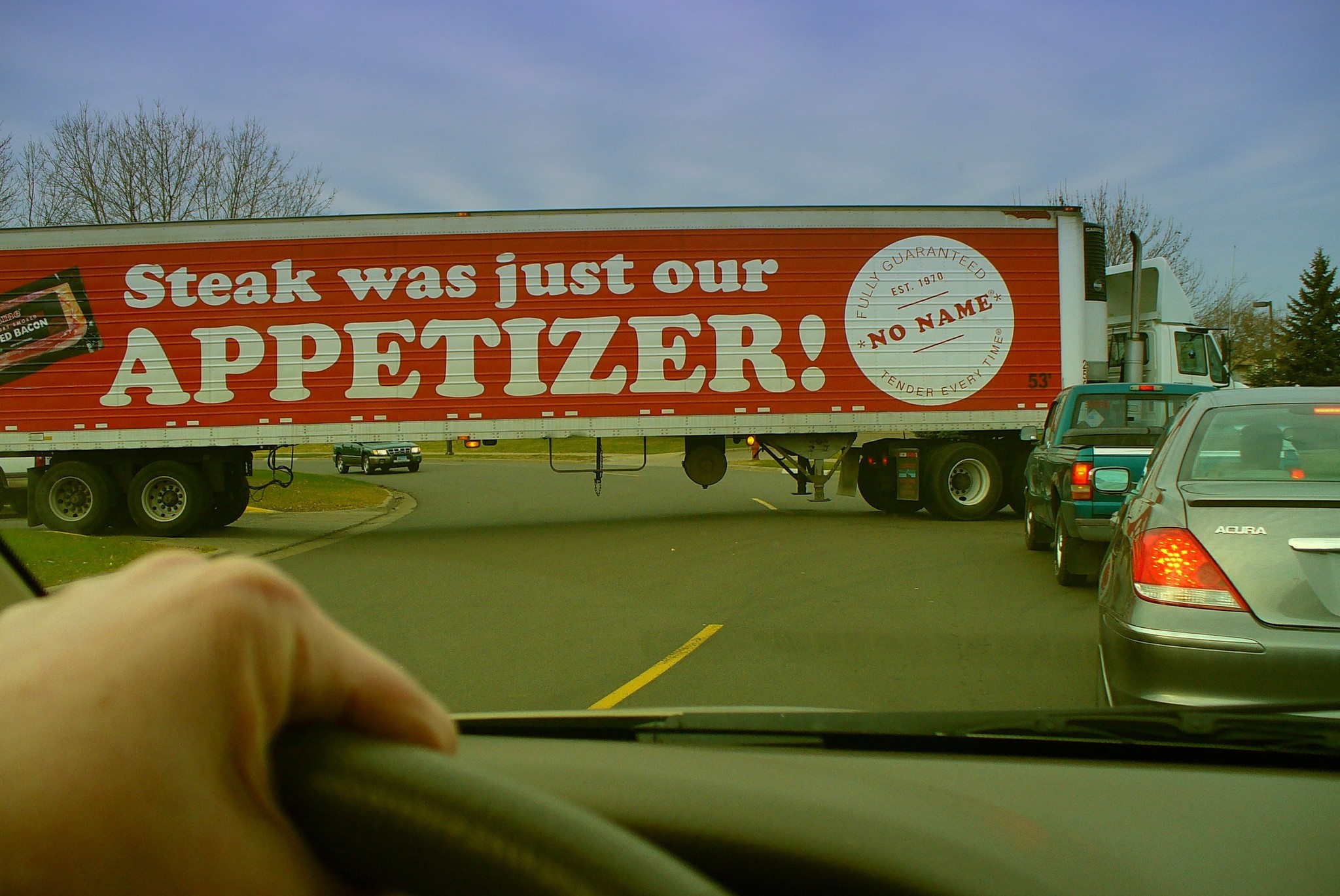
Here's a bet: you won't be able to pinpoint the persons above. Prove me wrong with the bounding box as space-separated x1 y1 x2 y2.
1237 421 1287 472
0 546 459 896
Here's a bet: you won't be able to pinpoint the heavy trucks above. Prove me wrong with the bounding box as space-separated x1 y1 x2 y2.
0 206 1296 538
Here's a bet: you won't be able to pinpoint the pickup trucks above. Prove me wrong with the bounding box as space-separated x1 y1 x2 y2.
1020 381 1300 586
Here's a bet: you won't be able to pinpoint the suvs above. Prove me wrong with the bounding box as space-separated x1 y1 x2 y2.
331 440 422 475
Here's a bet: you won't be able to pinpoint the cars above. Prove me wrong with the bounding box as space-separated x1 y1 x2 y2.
1094 386 1340 720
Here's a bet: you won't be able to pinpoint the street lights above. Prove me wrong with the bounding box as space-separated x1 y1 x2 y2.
1252 300 1275 387
